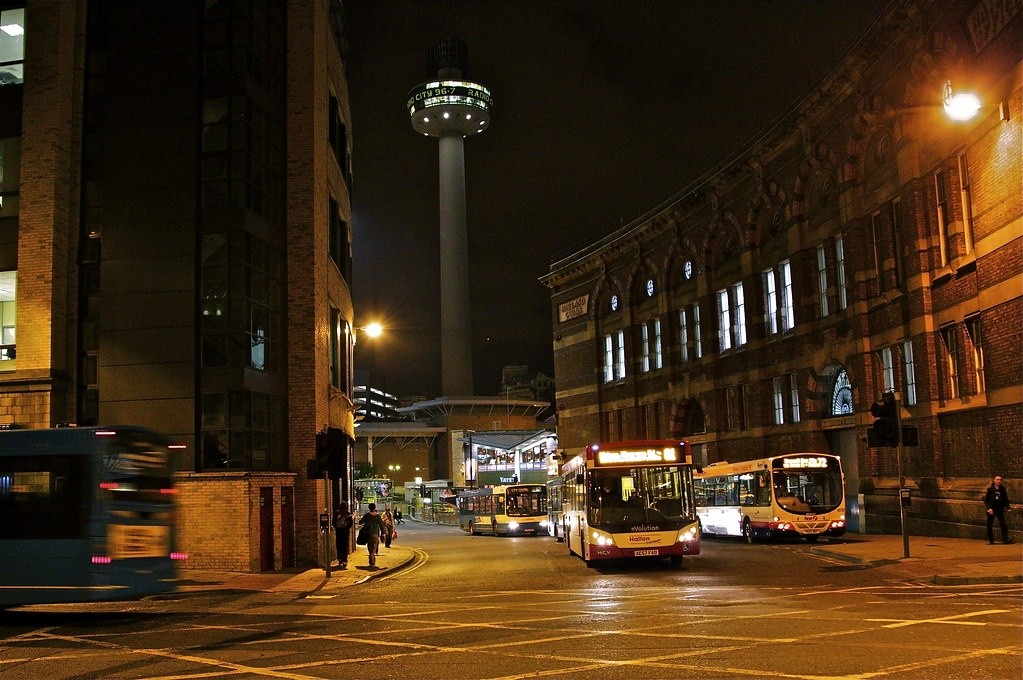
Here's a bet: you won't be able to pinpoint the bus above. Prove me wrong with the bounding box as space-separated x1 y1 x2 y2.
1 419 182 610
558 438 705 566
458 483 548 538
543 476 569 541
690 452 848 544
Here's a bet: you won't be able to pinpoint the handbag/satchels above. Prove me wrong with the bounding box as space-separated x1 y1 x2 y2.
391 532 397 540
337 515 348 528
357 527 367 544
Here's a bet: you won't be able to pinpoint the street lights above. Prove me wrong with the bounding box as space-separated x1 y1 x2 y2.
388 465 401 503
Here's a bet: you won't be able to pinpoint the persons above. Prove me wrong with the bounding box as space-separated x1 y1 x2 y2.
380 507 395 548
332 501 354 570
359 503 385 571
593 479 620 507
396 511 405 525
980 475 1012 545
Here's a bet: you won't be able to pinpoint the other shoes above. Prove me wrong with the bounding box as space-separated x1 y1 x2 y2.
339 561 347 568
987 540 994 544
1004 538 1013 544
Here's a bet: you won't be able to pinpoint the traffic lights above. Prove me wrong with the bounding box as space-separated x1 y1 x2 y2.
903 424 920 450
868 399 897 449
